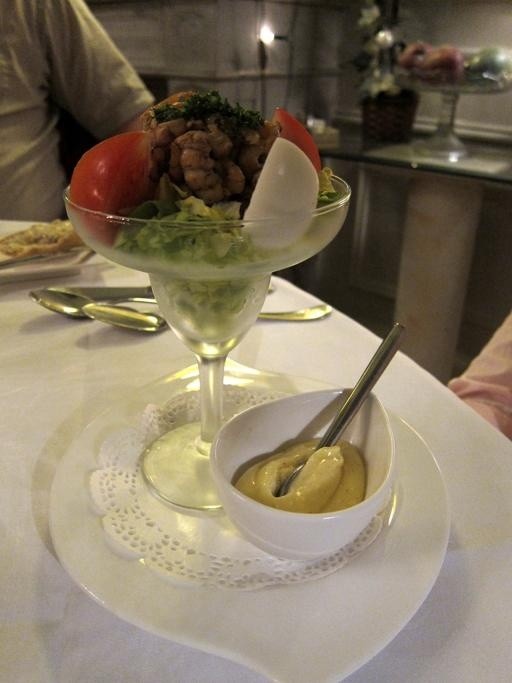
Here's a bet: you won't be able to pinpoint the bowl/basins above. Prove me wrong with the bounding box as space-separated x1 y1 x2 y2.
206 384 394 563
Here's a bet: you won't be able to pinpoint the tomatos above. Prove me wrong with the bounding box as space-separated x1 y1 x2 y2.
70 129 155 239
275 105 324 173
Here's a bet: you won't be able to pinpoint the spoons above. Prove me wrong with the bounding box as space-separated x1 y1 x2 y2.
79 302 336 335
31 286 280 317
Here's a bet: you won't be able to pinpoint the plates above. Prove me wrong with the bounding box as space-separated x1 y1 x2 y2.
46 345 450 683
1 214 90 283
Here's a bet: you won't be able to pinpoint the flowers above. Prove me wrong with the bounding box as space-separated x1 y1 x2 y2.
340 1 408 100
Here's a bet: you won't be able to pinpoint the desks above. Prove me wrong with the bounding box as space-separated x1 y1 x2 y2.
297 118 511 381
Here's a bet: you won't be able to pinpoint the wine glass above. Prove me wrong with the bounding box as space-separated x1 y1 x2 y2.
61 174 350 514
393 61 512 158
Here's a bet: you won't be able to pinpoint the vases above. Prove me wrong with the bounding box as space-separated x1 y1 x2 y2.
360 90 421 146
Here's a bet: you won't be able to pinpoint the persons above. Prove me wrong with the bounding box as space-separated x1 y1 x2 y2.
0 0 156 224
446 307 510 446
